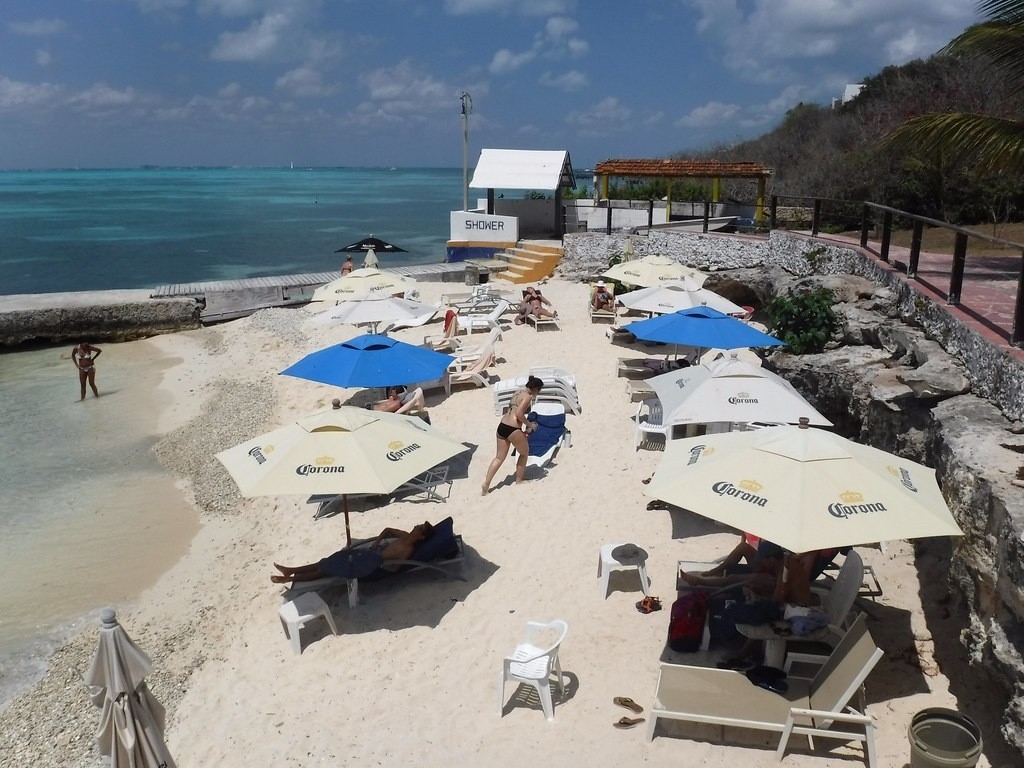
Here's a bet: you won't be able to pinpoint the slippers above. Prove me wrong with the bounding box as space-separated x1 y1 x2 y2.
613 716 646 728
613 697 644 714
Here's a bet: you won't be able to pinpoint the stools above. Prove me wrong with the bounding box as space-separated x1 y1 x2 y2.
277 591 338 656
597 544 650 600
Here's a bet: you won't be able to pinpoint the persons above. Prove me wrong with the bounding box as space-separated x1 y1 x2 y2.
524 287 557 319
270 521 436 583
365 386 405 412
680 546 843 670
590 281 617 312
71 339 102 401
340 256 353 276
481 376 544 496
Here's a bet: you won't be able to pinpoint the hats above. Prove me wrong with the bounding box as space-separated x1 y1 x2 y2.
594 280 607 288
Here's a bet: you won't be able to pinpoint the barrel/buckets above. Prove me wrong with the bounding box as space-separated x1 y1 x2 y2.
465 266 478 286
908 707 983 768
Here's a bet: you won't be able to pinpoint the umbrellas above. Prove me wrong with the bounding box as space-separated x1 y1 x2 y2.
646 417 966 621
309 263 417 301
615 276 747 317
303 287 441 334
600 236 711 292
277 328 457 410
214 398 471 551
85 607 178 768
619 301 788 366
643 350 834 433
334 233 409 269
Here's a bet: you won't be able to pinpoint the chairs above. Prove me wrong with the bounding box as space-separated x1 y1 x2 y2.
636 398 674 451
307 462 452 522
731 305 755 324
500 620 569 721
365 296 581 468
589 281 698 404
644 551 884 767
289 527 467 606
